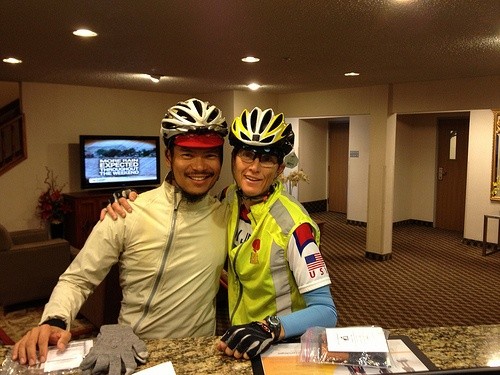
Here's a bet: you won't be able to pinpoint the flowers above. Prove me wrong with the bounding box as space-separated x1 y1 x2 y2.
36 168 73 228
281 168 310 196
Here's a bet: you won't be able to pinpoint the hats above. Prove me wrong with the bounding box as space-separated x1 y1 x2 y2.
173 133 224 148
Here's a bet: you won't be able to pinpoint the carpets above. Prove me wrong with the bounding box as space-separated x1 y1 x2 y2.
0 307 93 344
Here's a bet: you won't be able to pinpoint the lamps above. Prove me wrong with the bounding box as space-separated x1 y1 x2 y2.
150 75 160 83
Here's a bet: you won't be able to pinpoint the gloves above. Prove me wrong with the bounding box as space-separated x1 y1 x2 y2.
79 324 149 375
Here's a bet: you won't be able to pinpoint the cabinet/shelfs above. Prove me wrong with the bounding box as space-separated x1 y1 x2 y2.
70 189 158 252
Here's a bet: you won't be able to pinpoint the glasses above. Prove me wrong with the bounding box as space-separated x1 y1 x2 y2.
235 149 283 168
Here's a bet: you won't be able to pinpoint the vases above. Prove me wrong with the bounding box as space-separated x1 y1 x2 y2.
50 220 64 239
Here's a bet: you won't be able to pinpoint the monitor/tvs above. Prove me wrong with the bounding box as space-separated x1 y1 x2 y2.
79 135 160 189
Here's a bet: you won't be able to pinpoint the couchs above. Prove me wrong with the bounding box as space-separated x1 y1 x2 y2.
70 245 124 331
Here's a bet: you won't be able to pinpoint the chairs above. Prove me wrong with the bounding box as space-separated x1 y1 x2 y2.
0 225 72 311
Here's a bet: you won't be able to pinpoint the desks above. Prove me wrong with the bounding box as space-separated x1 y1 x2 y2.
0 325 500 375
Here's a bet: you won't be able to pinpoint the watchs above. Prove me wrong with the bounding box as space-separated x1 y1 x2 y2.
41 318 66 330
265 314 281 345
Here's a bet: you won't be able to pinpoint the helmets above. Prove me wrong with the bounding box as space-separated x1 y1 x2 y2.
227 107 295 155
159 97 229 145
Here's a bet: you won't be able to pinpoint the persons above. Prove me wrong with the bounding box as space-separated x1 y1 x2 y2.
100 104 337 361
10 98 234 365
